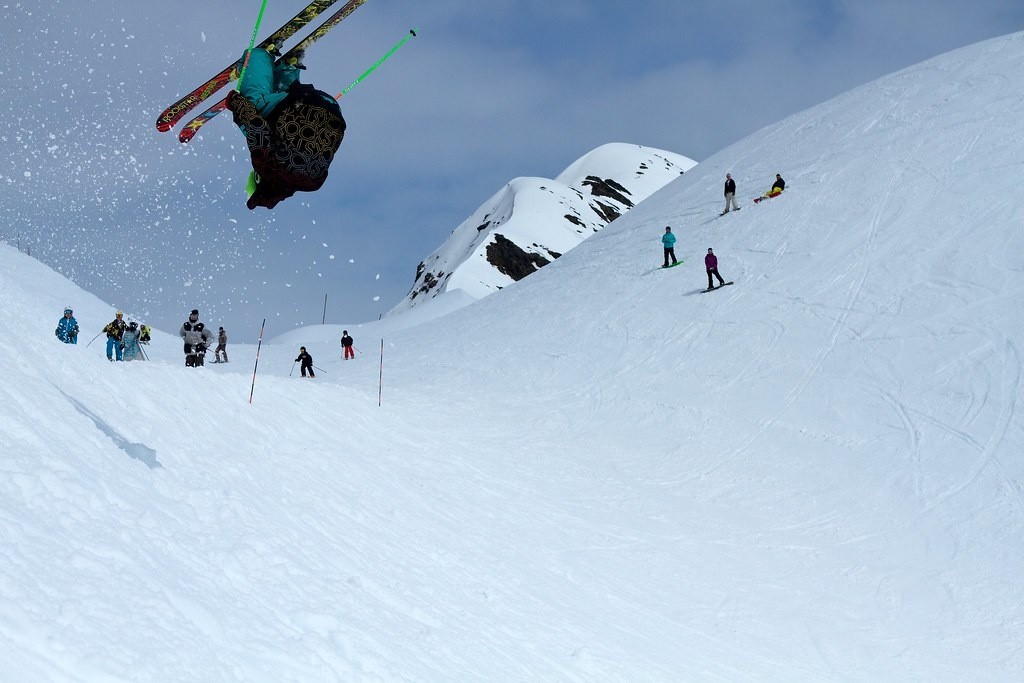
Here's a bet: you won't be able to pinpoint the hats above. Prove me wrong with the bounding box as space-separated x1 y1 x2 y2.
300 347 306 353
245 170 260 196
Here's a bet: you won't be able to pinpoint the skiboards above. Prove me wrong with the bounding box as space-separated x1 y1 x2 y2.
154 0 370 142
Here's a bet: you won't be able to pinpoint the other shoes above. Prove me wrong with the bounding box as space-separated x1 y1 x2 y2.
311 375 314 378
235 63 245 77
108 357 115 361
302 375 306 377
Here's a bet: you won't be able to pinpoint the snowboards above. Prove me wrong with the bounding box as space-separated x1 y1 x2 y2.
701 281 735 293
657 260 684 269
754 192 781 204
718 207 742 215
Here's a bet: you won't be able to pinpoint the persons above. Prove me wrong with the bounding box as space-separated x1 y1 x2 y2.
295 346 315 378
662 226 677 267
228 45 345 210
705 248 724 289
723 173 739 213
103 311 129 362
139 324 151 345
754 173 785 203
341 330 354 360
215 327 228 363
55 306 78 344
179 308 213 367
120 320 143 361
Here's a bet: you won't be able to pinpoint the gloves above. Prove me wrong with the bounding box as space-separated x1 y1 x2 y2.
225 90 238 111
125 327 130 332
311 364 313 366
295 359 298 362
130 326 136 332
119 344 125 350
69 331 75 337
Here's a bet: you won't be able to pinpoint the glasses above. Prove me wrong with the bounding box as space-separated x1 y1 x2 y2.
64 310 73 314
116 314 123 317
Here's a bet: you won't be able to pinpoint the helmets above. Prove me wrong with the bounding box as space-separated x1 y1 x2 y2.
63 306 74 315
130 322 138 330
116 311 123 318
189 310 199 323
219 327 223 331
